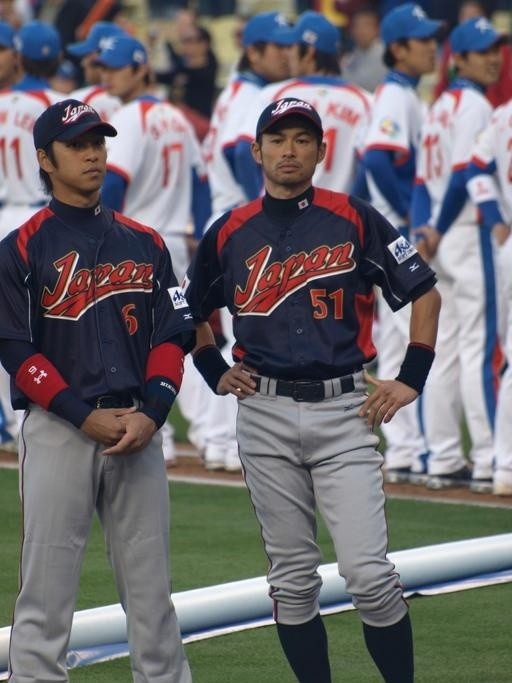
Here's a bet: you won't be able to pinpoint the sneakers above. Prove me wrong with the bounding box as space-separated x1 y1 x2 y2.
157 446 246 477
468 461 511 500
427 457 474 492
382 448 430 488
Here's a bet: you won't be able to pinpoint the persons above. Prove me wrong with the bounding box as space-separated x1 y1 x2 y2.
0 101 192 683
177 97 442 683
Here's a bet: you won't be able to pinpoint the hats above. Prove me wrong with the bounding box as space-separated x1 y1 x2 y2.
31 98 118 148
447 14 512 54
13 19 62 62
255 96 324 144
0 19 18 51
50 59 77 80
238 12 290 46
91 35 148 71
271 10 345 60
378 1 447 45
65 21 130 59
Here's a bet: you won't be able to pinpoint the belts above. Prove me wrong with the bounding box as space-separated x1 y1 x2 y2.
63 394 146 411
236 369 373 402
